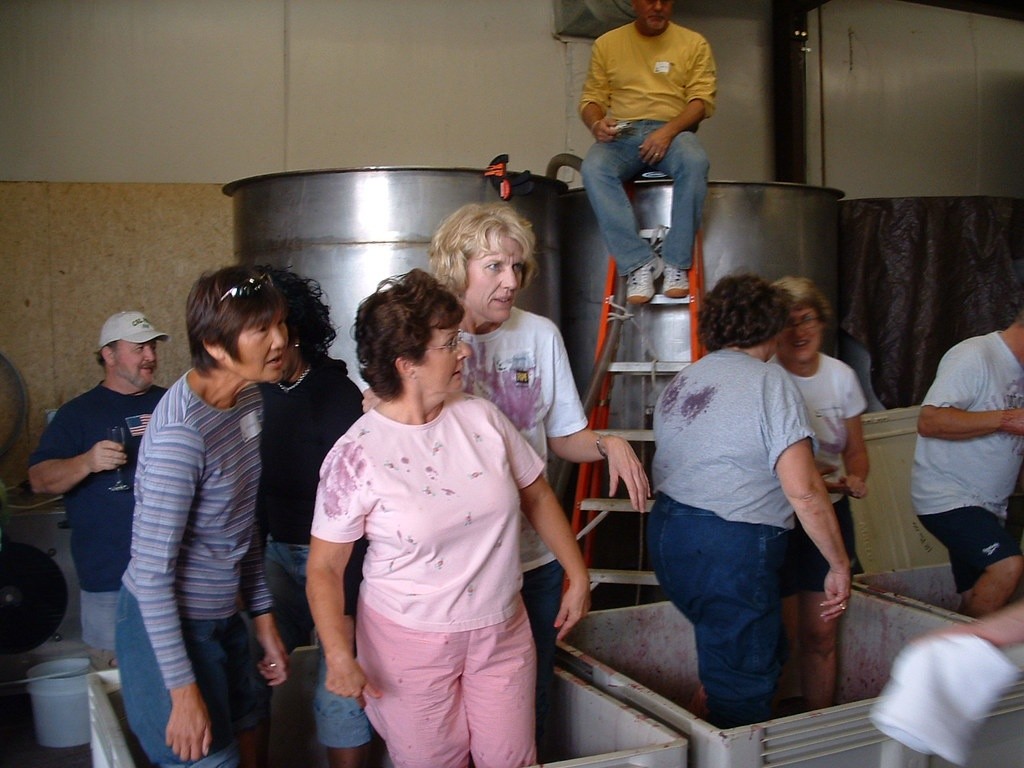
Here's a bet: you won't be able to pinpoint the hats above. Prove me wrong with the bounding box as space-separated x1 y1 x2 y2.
98 311 172 352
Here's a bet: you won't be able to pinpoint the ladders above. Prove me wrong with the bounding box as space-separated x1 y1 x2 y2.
562 229 706 603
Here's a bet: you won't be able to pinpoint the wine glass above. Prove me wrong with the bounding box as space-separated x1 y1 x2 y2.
107 425 131 491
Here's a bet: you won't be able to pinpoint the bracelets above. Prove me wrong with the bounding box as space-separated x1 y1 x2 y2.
590 120 601 132
596 434 610 459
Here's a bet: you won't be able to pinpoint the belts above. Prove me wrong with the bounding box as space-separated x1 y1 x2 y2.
654 498 718 517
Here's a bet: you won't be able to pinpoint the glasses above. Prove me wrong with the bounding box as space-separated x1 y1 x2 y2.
424 328 465 353
781 314 820 330
218 272 274 306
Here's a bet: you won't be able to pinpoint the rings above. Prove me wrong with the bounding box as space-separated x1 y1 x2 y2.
655 153 660 156
840 603 845 610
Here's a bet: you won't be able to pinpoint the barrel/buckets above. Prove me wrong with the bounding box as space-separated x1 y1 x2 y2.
26 657 93 749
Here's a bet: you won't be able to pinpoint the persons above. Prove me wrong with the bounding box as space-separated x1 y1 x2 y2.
919 598 1024 650
763 276 870 711
29 202 653 768
578 0 716 304
648 273 851 730
911 310 1024 617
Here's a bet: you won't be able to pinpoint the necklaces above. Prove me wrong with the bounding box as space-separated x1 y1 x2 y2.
276 363 312 393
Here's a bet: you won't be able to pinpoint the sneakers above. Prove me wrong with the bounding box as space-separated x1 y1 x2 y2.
662 262 691 298
626 257 665 305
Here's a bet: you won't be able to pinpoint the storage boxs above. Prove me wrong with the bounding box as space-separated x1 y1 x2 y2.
85 564 1024 768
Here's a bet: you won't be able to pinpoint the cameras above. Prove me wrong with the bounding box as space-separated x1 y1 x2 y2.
613 120 633 131
